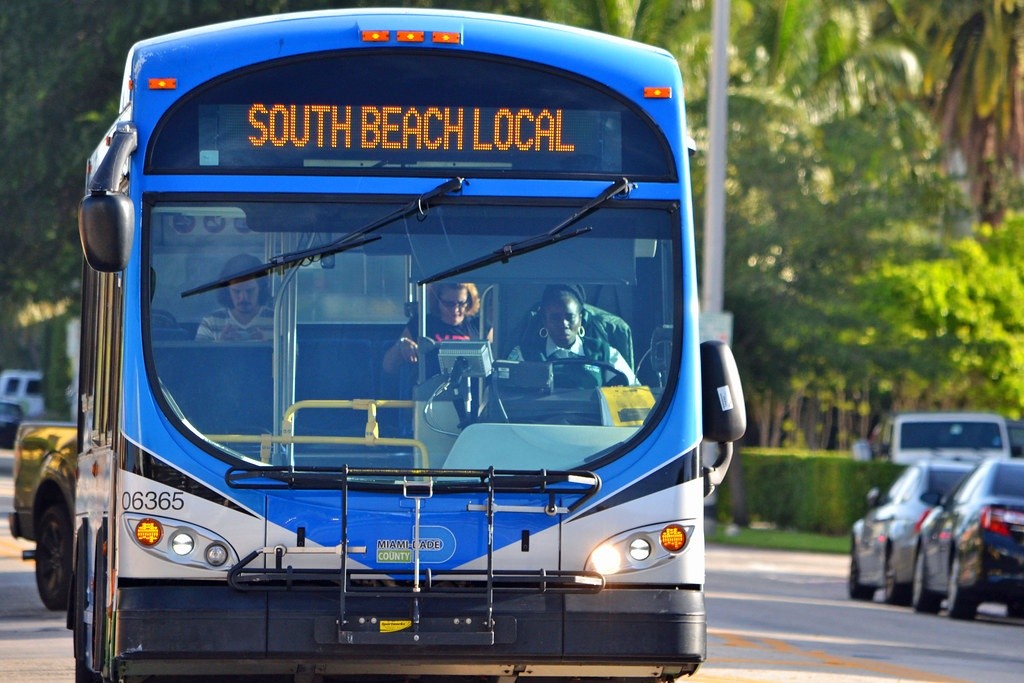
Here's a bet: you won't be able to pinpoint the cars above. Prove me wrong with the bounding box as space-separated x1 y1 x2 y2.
846 458 977 607
908 450 1023 621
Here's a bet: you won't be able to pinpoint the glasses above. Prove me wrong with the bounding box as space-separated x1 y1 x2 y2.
437 296 470 308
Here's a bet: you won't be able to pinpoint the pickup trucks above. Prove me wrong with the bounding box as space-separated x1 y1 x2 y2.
7 418 76 611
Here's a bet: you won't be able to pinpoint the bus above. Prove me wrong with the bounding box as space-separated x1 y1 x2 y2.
70 8 747 681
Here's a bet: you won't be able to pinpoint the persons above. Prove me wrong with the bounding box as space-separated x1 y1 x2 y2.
382 282 494 392
194 254 274 342
506 284 642 388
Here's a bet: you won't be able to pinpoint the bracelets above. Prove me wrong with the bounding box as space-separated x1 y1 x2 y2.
398 337 408 348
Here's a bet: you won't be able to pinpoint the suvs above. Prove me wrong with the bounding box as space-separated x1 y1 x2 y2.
856 410 1013 471
1 368 47 448
